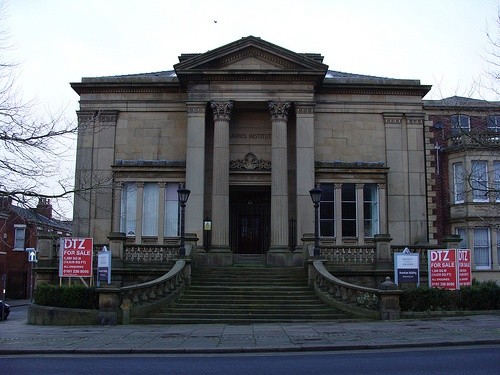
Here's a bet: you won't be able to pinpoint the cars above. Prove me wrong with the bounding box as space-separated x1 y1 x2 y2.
0 300 10 321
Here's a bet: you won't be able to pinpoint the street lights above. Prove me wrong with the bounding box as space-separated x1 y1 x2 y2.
175 184 190 259
309 184 322 256
